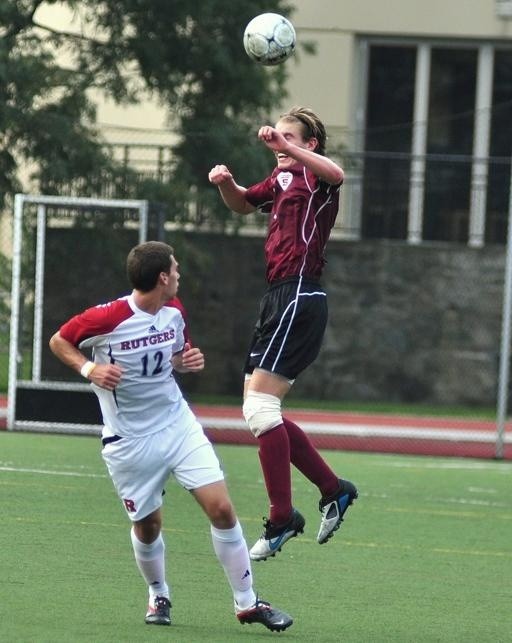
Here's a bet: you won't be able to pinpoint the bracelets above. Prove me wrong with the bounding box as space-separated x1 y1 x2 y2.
80 360 96 378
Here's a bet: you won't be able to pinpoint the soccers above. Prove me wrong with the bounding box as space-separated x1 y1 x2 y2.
243 13 297 65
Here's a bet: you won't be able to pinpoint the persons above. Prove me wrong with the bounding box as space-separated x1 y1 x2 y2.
47 240 294 634
208 104 358 563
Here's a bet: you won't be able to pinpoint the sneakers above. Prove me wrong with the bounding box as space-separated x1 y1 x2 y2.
145 586 173 625
317 478 358 544
233 591 293 632
249 507 306 561
